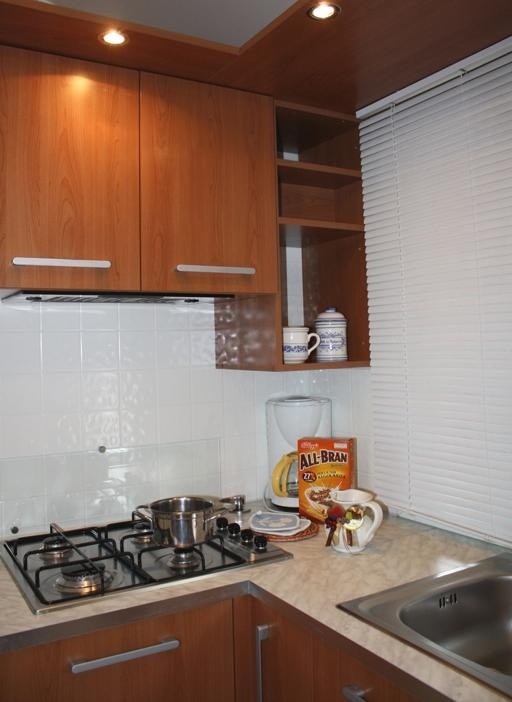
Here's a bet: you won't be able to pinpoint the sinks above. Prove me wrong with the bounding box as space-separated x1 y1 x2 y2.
336 551 512 697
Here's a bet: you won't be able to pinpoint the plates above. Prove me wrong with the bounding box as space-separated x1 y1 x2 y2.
305 486 337 513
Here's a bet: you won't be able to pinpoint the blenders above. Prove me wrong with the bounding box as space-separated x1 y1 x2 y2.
267 397 333 508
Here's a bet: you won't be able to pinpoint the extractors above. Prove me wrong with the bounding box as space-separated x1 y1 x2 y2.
1 290 234 304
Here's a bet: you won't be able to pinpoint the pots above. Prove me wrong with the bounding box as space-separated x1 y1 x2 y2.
134 496 236 547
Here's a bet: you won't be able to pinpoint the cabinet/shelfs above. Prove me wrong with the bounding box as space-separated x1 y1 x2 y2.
1 581 247 702
214 97 371 372
247 581 437 702
1 38 277 305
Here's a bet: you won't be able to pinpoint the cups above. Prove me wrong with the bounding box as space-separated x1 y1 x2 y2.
326 489 383 553
282 326 321 364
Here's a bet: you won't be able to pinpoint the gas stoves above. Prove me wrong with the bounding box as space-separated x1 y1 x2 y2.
0 515 292 616
